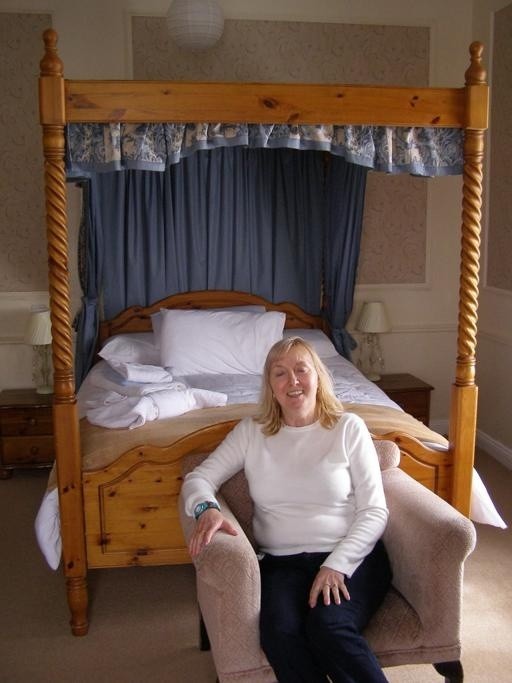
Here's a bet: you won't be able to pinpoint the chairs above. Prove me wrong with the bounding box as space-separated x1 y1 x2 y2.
176 436 479 681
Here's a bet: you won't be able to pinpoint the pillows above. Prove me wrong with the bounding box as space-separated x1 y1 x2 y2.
159 305 287 369
145 302 267 374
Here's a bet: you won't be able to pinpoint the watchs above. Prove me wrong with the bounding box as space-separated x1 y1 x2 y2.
194 501 221 519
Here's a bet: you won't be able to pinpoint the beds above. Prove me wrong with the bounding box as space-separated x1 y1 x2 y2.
37 26 491 638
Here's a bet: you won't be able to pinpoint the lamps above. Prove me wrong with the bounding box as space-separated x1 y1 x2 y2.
26 309 53 393
165 0 226 50
352 296 392 383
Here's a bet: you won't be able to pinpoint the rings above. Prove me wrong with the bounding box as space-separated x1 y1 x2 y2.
323 584 331 586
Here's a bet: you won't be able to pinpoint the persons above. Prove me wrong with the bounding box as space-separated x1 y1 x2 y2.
183 336 393 683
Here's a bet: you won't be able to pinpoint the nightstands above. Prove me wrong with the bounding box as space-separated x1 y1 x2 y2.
372 369 438 429
2 385 57 477
